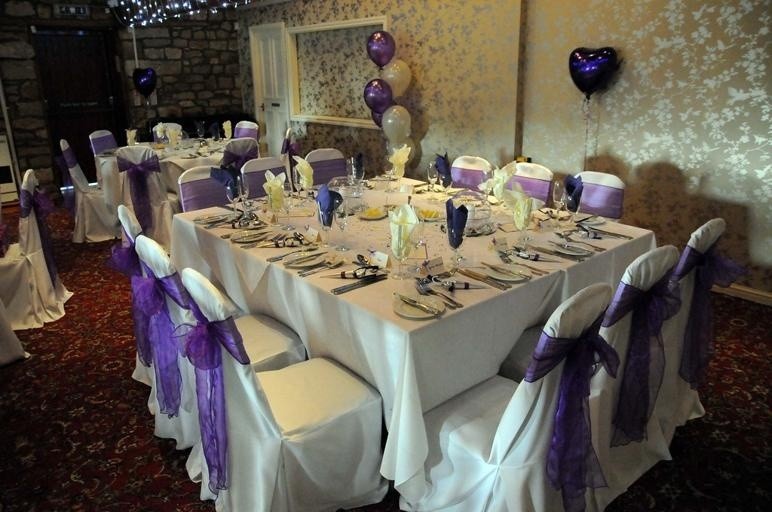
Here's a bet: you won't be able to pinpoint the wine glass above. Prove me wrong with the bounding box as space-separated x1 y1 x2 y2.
239 183 251 220
157 126 165 145
334 199 350 250
392 222 411 279
428 162 437 200
227 181 242 220
513 198 536 249
346 153 365 197
565 193 581 225
197 128 203 140
293 167 305 207
440 180 452 203
553 178 564 227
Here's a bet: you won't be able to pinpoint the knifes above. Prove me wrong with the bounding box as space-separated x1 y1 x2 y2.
400 295 441 314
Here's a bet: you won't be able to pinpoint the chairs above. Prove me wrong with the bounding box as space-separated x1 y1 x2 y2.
135 234 306 450
672 217 727 391
503 245 681 497
451 156 492 194
1 168 74 330
180 267 389 511
574 171 626 223
399 282 615 512
505 162 554 208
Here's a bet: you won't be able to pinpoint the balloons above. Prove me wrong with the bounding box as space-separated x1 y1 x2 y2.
366 30 396 71
133 67 156 98
371 99 400 128
382 105 411 145
364 78 391 116
569 47 618 99
382 60 411 100
386 137 415 168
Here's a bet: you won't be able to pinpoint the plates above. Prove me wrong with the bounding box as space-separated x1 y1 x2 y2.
574 214 605 224
416 207 440 221
555 242 593 256
195 215 227 223
230 230 265 242
282 252 324 267
181 154 197 158
393 296 445 317
487 264 531 281
361 210 388 220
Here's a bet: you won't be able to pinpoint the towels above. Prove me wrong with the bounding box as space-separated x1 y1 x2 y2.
446 199 466 250
564 175 583 215
387 205 419 259
477 162 518 203
503 183 532 229
388 145 411 170
434 152 452 188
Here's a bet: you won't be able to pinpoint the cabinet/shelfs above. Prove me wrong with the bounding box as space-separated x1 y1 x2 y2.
0 85 24 207
